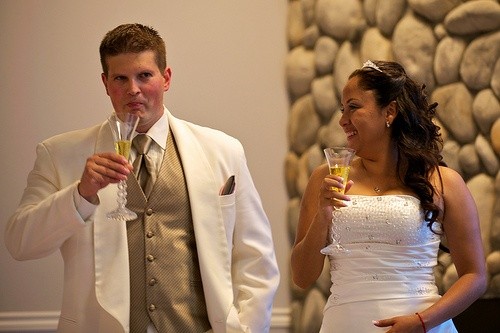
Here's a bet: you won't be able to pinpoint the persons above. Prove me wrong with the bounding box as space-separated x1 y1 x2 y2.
290 59 488 333
3 24 281 332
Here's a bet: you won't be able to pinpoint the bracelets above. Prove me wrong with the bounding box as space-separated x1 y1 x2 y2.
416 312 427 333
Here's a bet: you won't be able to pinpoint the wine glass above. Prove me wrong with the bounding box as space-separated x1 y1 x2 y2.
106 112 140 221
320 146 356 256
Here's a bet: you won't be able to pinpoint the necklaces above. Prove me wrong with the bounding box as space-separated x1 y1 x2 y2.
370 184 385 194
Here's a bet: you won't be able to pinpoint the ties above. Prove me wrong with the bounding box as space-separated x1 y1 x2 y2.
129 133 158 200
